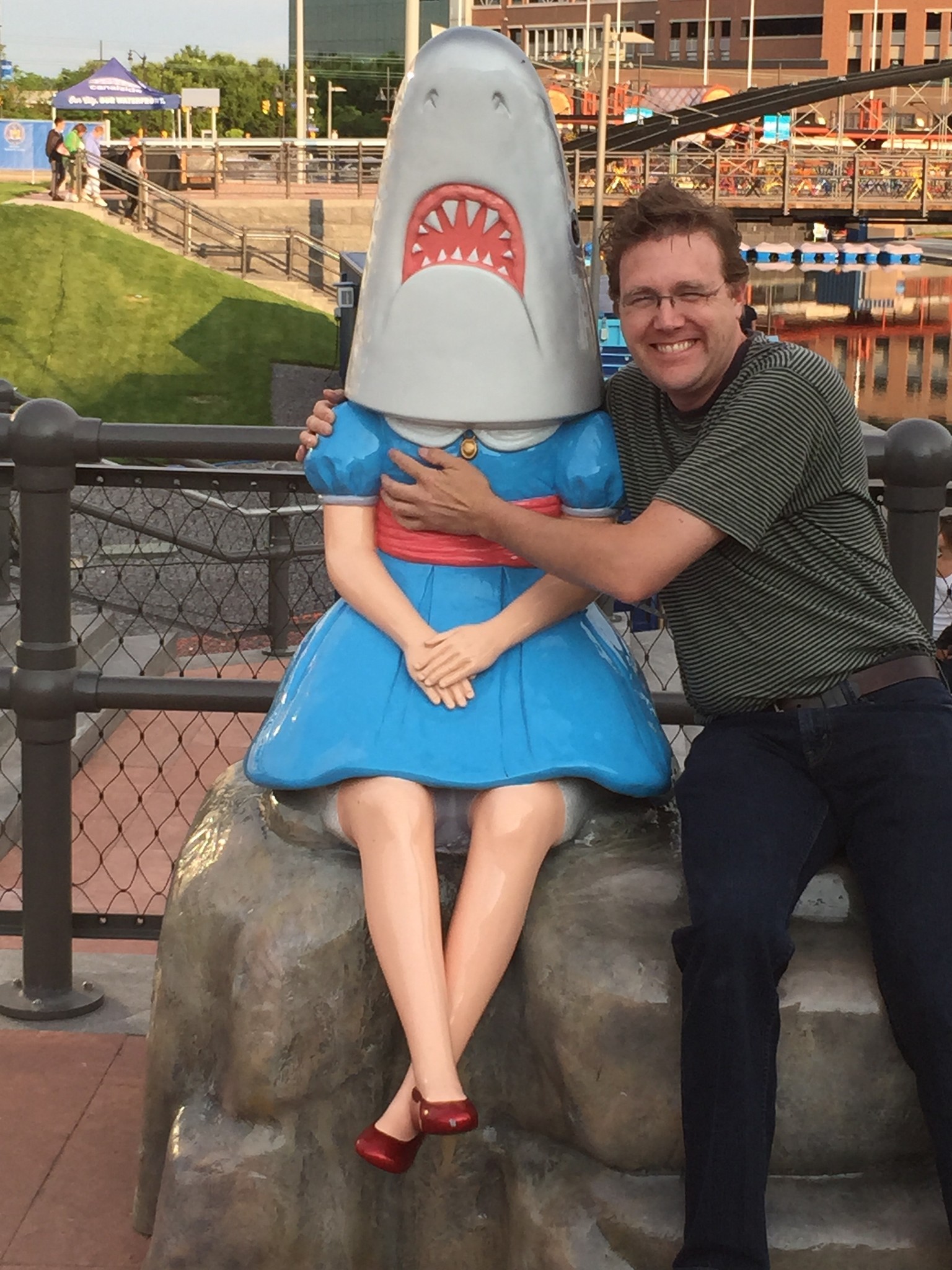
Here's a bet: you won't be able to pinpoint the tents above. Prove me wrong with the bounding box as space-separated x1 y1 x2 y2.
52 58 182 140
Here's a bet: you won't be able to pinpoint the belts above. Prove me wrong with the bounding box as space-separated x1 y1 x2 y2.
773 655 940 713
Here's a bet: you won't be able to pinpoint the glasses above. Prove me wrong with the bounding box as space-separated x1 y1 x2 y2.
616 275 730 319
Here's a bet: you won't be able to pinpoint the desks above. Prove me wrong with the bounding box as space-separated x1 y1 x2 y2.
82 149 181 192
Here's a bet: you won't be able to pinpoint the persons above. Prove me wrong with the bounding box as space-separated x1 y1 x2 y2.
297 181 951 1268
48 118 150 228
234 27 678 1185
560 126 952 201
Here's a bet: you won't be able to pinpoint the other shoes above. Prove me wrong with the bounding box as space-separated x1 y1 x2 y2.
95 198 107 206
82 191 95 201
66 192 78 202
411 1085 477 1133
356 1118 424 1172
48 191 64 201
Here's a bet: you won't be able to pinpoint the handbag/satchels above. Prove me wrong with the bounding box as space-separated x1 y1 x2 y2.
56 132 76 157
